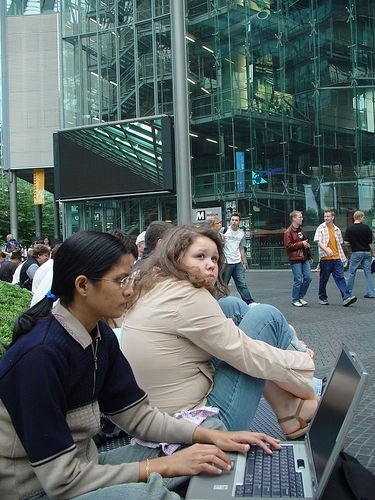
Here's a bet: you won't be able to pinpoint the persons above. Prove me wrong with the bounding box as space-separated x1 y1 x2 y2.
284 211 314 306
1 231 284 500
0 220 176 319
207 216 224 241
217 213 260 306
343 210 375 298
119 225 325 441
314 209 357 307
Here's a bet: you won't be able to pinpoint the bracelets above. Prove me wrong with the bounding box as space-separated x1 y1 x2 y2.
146 459 150 481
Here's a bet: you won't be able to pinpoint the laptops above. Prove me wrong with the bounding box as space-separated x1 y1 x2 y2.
186 342 368 500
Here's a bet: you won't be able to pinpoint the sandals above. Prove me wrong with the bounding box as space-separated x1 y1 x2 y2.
277 399 320 439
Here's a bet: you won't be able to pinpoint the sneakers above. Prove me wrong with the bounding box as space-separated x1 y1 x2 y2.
343 295 356 307
291 299 308 307
317 298 330 305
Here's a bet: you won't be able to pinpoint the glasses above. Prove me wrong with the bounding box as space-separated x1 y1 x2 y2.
88 272 137 289
